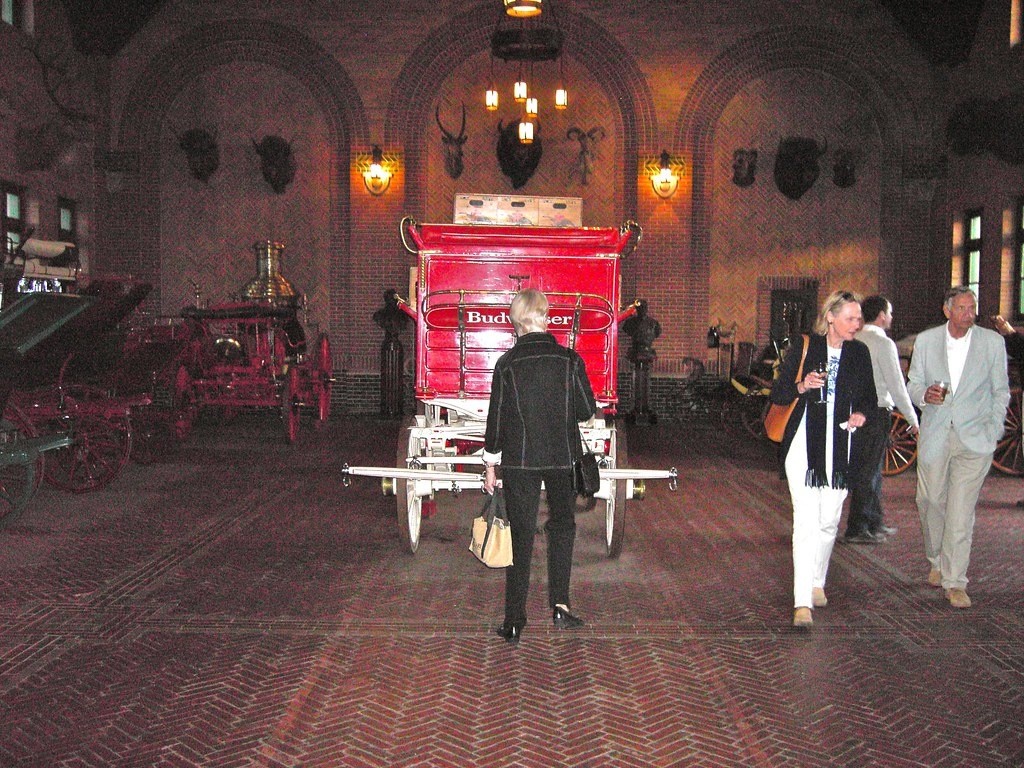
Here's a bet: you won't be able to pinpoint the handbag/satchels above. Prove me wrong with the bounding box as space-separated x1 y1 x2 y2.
468 485 514 568
572 452 601 495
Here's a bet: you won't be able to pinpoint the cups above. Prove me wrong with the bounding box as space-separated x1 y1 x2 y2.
935 380 950 404
16 277 62 293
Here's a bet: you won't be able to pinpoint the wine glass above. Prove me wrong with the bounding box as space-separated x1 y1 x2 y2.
812 361 830 404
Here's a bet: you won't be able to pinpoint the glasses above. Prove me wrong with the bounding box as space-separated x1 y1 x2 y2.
830 292 852 310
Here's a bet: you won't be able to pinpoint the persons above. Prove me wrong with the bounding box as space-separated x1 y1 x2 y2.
622 300 662 346
485 289 597 643
846 295 920 543
373 288 408 334
770 291 879 626
905 287 1011 608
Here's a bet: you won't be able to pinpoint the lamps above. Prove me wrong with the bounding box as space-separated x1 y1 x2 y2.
363 146 393 195
486 0 568 144
650 149 680 198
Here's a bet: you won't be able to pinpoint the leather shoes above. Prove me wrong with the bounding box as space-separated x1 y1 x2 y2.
497 623 519 644
553 606 583 628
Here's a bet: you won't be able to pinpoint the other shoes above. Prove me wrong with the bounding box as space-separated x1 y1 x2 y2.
848 531 886 543
764 334 809 443
930 570 941 586
792 608 813 627
811 587 828 607
874 526 895 535
945 586 971 607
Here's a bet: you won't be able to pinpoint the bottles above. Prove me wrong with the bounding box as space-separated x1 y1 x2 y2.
194 283 202 309
